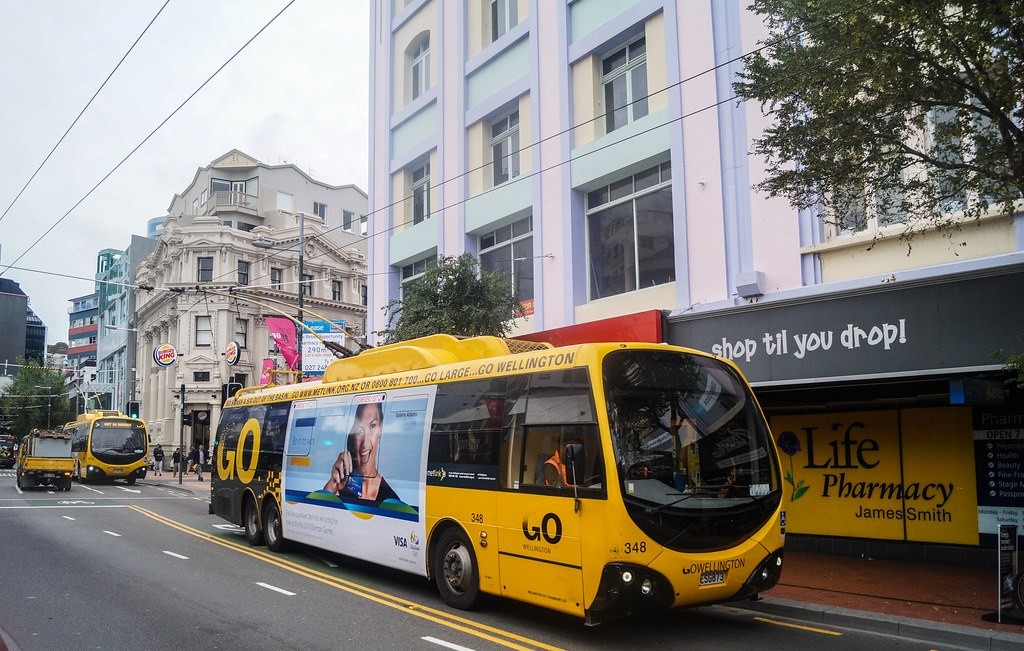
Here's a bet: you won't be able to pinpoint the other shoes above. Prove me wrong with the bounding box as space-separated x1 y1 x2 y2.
194 472 198 474
173 476 175 477
184 473 188 475
198 476 203 481
154 473 156 476
159 473 161 476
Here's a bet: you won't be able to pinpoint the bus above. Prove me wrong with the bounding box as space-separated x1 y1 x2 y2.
62 383 151 486
207 291 788 628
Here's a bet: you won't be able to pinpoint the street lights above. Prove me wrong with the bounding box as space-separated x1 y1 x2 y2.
252 213 306 382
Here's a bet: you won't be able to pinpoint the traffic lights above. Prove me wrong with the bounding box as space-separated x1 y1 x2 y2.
128 400 141 419
226 382 243 400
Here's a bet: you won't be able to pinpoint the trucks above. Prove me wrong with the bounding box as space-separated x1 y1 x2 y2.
13 433 76 492
0 434 19 470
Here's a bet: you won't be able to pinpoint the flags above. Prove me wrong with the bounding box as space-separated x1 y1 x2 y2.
265 317 301 371
260 360 274 384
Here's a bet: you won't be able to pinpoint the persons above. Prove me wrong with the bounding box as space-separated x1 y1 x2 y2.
542 450 576 487
153 443 210 482
322 403 401 508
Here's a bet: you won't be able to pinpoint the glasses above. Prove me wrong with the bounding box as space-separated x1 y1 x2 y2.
199 448 203 450
